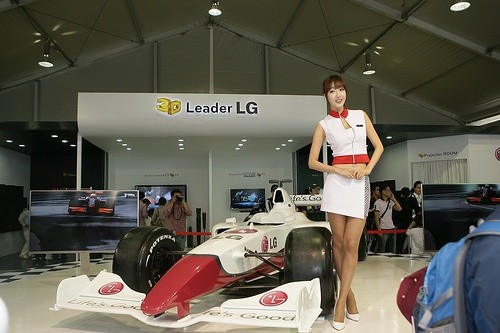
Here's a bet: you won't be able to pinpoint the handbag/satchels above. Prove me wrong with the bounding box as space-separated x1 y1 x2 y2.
372 217 380 231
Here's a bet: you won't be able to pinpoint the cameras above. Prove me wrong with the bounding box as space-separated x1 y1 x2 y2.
176 195 183 202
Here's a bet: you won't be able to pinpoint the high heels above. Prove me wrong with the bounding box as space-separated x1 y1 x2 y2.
345 287 360 322
332 299 346 332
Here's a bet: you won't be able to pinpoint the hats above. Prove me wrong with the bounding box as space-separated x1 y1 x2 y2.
309 183 320 191
271 184 278 192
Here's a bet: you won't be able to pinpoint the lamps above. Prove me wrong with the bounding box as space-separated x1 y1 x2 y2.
208 0 222 17
448 0 471 12
38 37 54 67
362 52 376 75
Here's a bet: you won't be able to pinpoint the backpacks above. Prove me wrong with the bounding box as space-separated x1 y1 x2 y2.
410 220 500 333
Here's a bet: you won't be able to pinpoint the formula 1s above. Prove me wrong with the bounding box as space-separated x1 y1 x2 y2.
466 184 500 206
68 190 115 216
49 177 369 333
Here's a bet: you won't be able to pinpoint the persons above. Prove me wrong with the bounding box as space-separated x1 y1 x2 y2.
139 189 193 250
465 204 500 333
307 75 383 331
267 181 423 254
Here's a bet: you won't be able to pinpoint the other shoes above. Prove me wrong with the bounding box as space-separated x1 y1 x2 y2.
18 254 29 258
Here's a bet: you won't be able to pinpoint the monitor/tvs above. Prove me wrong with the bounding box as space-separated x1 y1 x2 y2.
421 183 500 252
28 189 140 254
230 188 265 208
135 185 187 215
